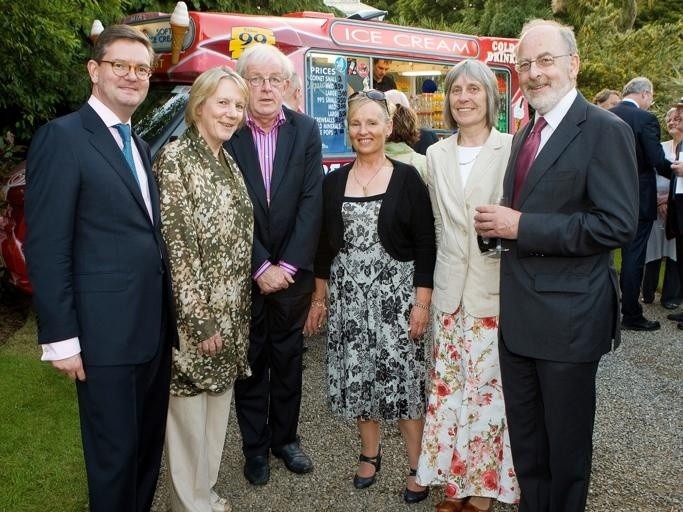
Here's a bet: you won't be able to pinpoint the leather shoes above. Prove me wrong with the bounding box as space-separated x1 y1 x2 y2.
663 301 678 309
271 442 314 474
621 317 661 331
244 455 269 485
668 314 683 321
677 323 683 329
437 500 465 512
461 496 493 512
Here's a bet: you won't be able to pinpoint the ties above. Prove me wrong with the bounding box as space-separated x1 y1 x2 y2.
112 123 140 187
511 116 547 208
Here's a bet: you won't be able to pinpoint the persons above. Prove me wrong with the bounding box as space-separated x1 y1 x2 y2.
231 40 326 489
148 63 260 512
23 22 183 512
282 55 682 512
471 16 642 512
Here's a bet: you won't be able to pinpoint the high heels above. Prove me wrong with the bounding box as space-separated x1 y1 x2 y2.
404 467 429 504
354 444 383 489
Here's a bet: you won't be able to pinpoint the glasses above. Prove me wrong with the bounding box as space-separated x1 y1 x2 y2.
645 93 656 106
514 54 572 73
243 74 288 87
348 90 390 116
100 58 153 81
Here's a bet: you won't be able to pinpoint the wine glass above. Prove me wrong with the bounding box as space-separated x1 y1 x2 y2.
489 196 509 252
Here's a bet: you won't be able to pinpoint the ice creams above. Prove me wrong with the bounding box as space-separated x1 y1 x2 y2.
513 96 526 132
90 18 105 49
168 1 190 64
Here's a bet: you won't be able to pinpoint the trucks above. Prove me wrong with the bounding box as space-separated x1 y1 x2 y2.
0 5 530 294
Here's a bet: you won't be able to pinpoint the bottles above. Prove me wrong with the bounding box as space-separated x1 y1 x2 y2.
408 91 445 130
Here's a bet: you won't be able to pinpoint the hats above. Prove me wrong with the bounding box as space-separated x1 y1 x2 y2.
669 98 683 106
382 89 410 113
